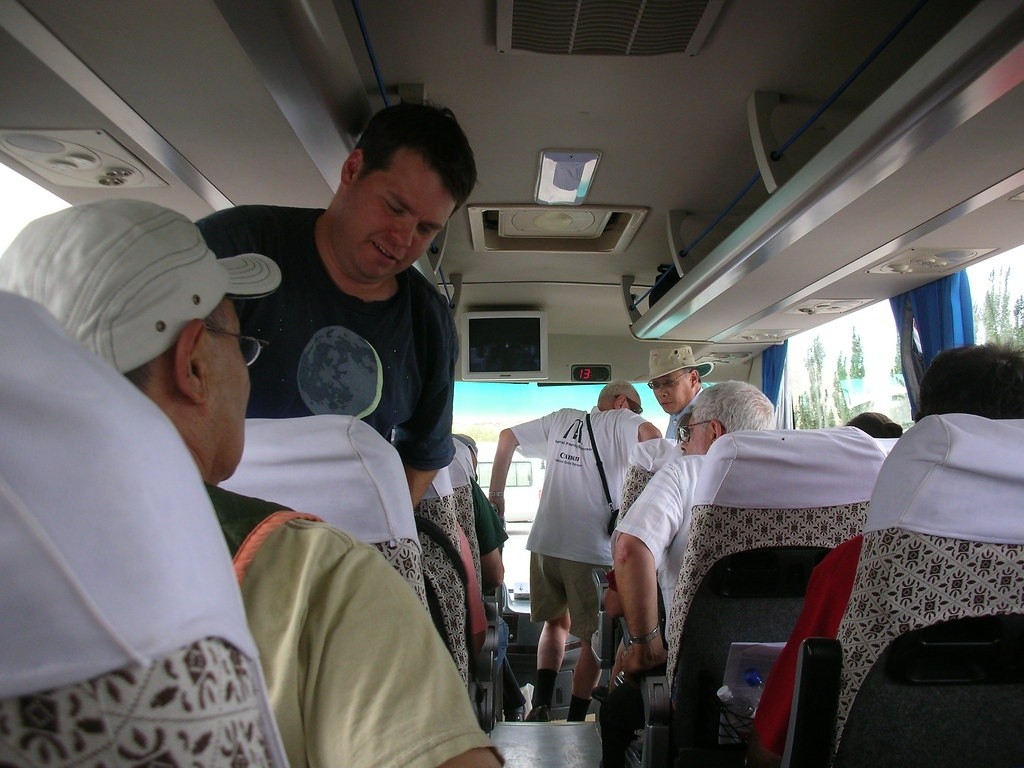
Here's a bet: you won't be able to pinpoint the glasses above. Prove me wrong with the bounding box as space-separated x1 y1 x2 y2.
647 369 696 389
677 420 725 443
614 394 643 414
203 323 262 366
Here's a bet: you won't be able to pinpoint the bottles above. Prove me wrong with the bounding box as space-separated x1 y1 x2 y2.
716 685 757 746
744 669 767 706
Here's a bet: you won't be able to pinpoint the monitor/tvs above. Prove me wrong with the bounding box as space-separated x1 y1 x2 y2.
461 311 550 380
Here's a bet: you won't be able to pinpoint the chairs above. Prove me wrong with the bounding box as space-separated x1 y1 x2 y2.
0 288 478 768
778 413 1024 768
590 435 682 669
620 427 888 768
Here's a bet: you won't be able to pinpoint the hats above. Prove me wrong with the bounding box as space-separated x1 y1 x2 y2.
634 345 714 382
1 199 283 376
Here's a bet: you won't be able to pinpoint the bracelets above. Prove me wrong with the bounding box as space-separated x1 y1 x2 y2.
627 623 662 644
488 490 504 498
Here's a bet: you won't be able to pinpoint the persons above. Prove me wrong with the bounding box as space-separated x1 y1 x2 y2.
847 410 903 439
747 343 1023 768
197 101 477 513
0 197 507 768
456 432 502 585
488 382 659 722
647 345 715 439
599 381 776 768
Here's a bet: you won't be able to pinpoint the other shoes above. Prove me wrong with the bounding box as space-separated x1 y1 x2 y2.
504 710 521 722
525 704 551 722
